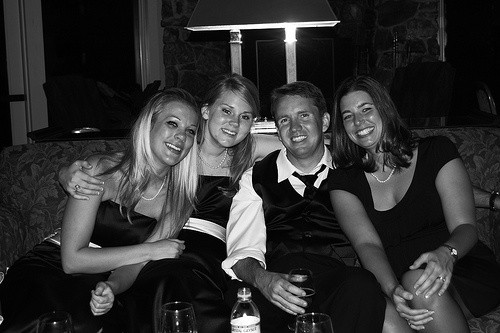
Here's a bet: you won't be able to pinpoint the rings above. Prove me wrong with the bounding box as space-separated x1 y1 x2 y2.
437 275 446 283
74 186 79 192
408 322 411 326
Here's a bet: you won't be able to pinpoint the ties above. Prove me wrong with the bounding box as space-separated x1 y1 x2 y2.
291 164 327 200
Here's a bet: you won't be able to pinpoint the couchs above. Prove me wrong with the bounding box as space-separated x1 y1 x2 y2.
0 124 500 333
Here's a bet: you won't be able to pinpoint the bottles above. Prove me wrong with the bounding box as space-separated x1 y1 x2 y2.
230 287 261 333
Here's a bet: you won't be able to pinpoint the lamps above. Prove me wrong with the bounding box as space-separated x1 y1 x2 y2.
186 0 340 32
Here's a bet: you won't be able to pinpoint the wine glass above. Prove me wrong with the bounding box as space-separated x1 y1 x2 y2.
288 269 315 331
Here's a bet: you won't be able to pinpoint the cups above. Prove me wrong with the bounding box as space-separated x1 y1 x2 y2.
36 310 74 333
156 301 198 333
295 313 334 333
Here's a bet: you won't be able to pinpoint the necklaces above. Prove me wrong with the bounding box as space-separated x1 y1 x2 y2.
366 143 407 184
195 147 229 168
115 154 169 200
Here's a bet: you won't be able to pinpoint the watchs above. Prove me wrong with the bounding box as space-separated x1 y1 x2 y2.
438 243 460 263
488 190 499 210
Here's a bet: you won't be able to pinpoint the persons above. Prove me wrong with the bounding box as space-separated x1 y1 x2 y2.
0 87 201 333
329 78 500 333
55 67 333 333
224 77 500 333
